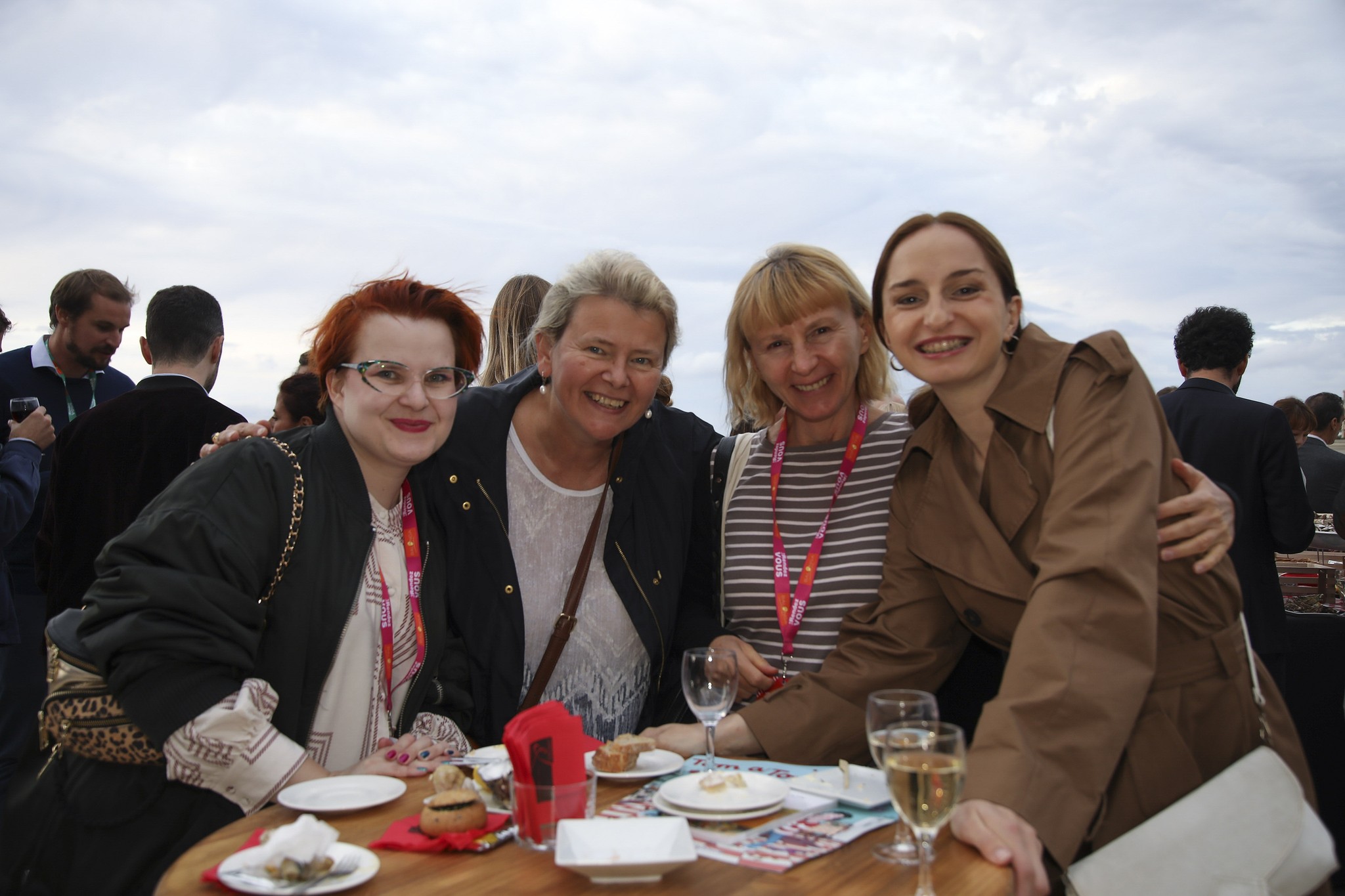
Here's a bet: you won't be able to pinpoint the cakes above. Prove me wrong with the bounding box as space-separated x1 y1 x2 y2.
694 771 749 794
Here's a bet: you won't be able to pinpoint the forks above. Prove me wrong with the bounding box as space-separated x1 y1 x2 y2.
289 852 363 894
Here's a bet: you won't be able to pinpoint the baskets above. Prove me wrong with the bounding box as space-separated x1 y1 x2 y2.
1274 550 1345 578
1275 562 1336 605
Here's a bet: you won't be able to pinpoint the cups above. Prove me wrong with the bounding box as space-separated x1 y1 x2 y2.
506 770 597 851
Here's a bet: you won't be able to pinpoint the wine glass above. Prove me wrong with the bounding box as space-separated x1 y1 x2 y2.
681 647 738 771
866 686 939 865
881 718 967 895
10 396 45 456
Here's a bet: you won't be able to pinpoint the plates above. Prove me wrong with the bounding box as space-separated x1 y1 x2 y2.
555 816 698 884
216 841 380 895
653 771 793 823
278 774 407 815
464 744 511 771
582 748 686 782
787 764 891 811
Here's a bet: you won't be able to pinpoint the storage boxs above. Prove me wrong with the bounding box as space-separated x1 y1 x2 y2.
1274 562 1336 603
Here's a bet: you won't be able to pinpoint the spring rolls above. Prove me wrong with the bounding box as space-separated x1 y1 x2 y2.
260 814 340 883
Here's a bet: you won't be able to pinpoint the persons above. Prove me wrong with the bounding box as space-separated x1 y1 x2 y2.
0 309 56 786
600 211 1334 896
1295 391 1345 547
28 273 478 896
34 284 248 656
1155 306 1316 696
198 252 725 750
479 273 553 389
1273 396 1314 490
677 245 916 771
297 349 320 372
1 268 138 766
268 372 327 432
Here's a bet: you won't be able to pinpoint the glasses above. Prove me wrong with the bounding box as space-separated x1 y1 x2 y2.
337 360 476 401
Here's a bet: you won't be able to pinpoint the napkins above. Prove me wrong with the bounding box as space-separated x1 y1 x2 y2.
368 811 511 851
197 826 269 895
502 699 607 845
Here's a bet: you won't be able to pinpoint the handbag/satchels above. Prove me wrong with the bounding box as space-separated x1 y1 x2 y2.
1062 744 1339 896
39 606 167 769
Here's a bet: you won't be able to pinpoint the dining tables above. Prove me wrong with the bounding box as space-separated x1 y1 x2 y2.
154 764 1018 895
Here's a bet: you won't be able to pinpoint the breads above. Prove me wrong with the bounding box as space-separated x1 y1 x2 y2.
591 733 655 773
